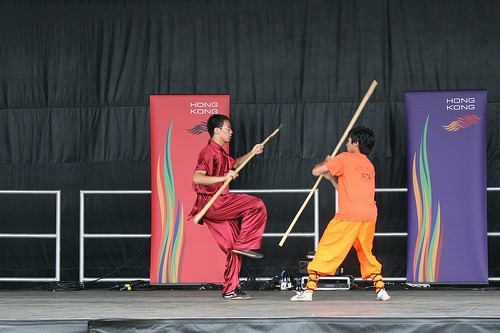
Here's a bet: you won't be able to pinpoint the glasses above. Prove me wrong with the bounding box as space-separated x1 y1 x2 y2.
219 127 231 132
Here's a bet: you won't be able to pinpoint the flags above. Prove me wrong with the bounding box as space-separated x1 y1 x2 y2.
405 90 489 285
149 95 230 285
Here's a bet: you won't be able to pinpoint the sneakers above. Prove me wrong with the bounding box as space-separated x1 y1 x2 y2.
222 288 251 300
290 290 312 301
377 288 390 301
232 250 264 258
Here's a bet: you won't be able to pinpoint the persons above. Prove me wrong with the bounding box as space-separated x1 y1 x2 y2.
188 115 267 301
290 127 390 301
